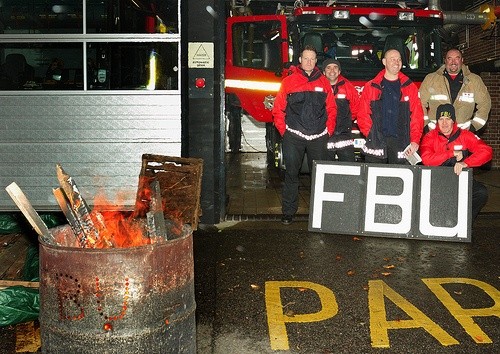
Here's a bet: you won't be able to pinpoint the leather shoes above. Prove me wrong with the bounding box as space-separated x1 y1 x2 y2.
281 214 292 224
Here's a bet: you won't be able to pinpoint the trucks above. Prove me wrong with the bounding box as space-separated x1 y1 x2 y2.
224 0 444 176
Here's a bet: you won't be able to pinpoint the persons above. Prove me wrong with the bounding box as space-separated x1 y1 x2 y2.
271 46 337 225
319 59 372 162
418 47 492 132
357 48 424 165
417 104 496 227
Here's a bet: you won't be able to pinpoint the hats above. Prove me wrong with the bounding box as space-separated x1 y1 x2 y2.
322 58 341 71
436 103 456 121
